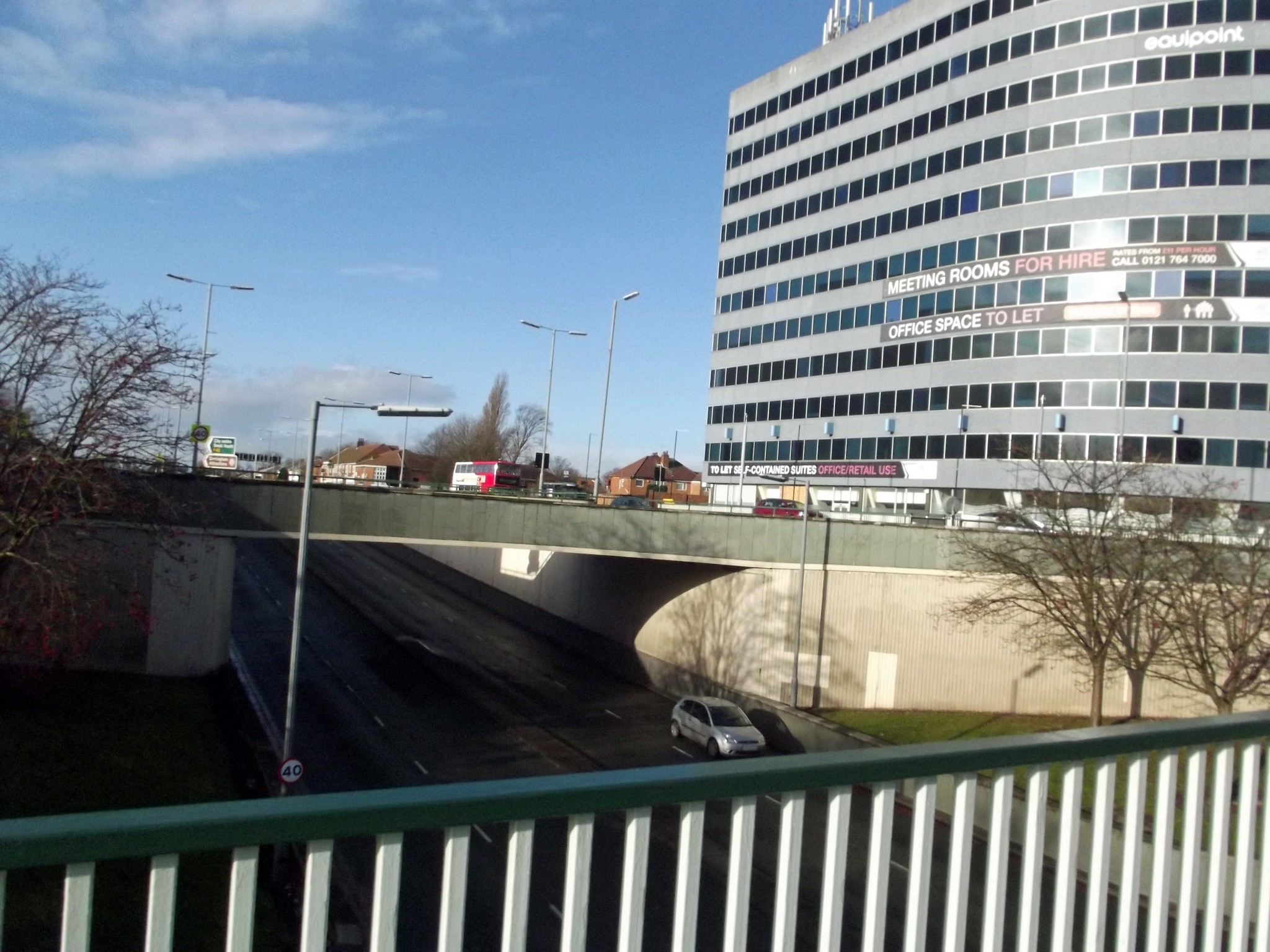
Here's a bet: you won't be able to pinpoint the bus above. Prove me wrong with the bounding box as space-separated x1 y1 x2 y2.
542 481 578 500
451 457 525 496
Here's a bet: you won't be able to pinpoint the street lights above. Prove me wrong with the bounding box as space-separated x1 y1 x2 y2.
594 291 640 503
1118 291 1129 530
656 463 662 502
759 471 809 707
388 370 432 489
522 319 588 496
167 272 255 473
283 396 455 795
951 404 982 527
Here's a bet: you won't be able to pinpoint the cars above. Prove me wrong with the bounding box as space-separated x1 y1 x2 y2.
672 692 765 758
973 508 1043 534
753 499 825 520
609 496 654 510
